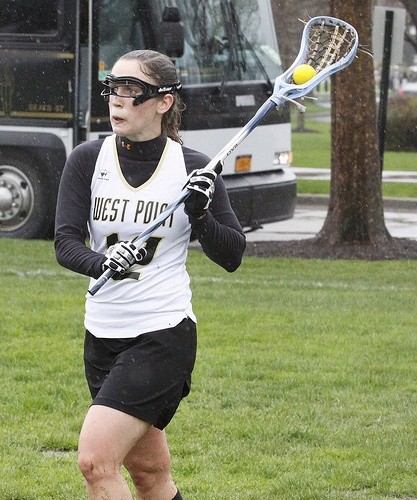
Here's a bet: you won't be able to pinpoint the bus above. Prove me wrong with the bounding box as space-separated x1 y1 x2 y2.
0 0 298 241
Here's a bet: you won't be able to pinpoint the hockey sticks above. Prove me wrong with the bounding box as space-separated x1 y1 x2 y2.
86 15 359 296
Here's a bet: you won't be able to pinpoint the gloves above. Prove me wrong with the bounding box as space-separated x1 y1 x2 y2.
100 241 148 274
182 161 223 223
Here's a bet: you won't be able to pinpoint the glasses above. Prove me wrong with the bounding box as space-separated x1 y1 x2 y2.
100 75 159 107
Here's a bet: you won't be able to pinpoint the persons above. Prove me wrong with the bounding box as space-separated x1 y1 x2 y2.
55 48 246 500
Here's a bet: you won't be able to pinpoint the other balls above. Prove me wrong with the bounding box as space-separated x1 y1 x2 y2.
292 64 317 86
